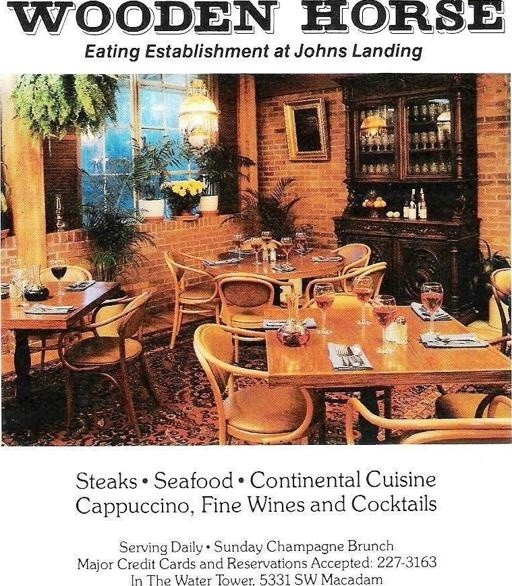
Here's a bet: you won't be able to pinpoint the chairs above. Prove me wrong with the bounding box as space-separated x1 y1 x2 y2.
57 288 167 443
27 266 94 371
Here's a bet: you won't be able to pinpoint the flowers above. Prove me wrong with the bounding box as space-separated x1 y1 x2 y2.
160 180 208 210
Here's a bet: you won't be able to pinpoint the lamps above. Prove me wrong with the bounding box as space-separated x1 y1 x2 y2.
177 74 219 148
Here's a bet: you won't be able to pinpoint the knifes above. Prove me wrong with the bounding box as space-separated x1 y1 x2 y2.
345 347 361 367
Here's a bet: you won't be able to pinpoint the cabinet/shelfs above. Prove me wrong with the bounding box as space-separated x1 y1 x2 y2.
331 73 487 325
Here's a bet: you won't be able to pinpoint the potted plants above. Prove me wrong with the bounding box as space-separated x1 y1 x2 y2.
184 144 255 216
108 136 183 219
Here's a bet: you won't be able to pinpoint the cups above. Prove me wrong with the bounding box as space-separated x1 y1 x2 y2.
358 161 451 174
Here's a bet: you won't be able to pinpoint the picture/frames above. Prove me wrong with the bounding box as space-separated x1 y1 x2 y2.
282 98 331 161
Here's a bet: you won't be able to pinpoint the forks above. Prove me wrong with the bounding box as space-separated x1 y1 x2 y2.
335 346 349 370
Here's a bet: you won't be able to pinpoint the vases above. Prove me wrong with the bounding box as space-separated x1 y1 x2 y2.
172 205 192 217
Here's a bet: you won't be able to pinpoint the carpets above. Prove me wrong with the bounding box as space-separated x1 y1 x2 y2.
2 314 512 445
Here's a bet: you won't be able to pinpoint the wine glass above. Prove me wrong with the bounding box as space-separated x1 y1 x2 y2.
48 257 68 298
233 230 309 269
358 101 452 151
311 275 445 356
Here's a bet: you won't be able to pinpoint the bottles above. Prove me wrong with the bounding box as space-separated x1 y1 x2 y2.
275 291 311 346
402 188 429 221
23 263 49 300
386 316 408 345
